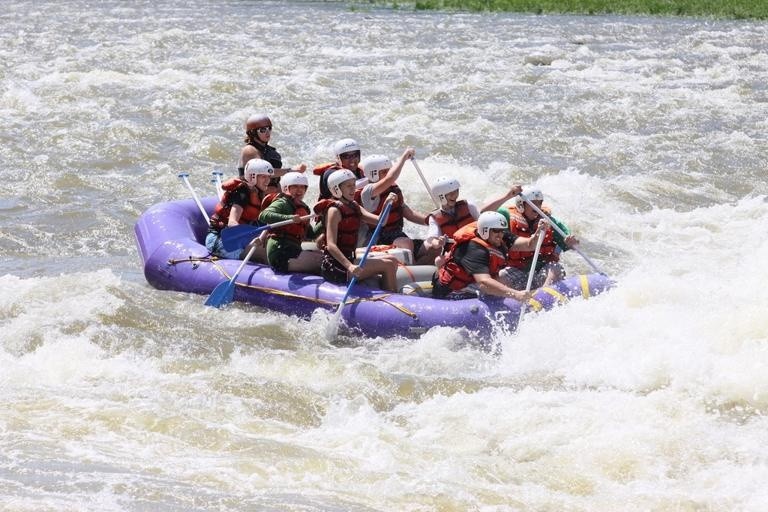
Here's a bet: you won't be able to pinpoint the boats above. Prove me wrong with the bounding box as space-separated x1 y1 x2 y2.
133 194 617 355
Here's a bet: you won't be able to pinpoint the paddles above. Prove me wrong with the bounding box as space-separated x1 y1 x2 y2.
221 214 315 253
324 200 393 336
204 223 268 308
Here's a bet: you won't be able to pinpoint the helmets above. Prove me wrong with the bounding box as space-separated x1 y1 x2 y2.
243 158 274 186
245 114 271 131
363 154 392 183
278 172 308 194
514 186 543 214
326 168 355 199
334 138 360 166
431 177 460 206
477 211 508 241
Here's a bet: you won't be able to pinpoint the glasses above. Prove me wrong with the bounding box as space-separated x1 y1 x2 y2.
341 153 357 159
492 228 504 233
256 126 271 133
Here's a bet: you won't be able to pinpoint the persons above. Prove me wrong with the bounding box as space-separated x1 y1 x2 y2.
205 114 581 302
